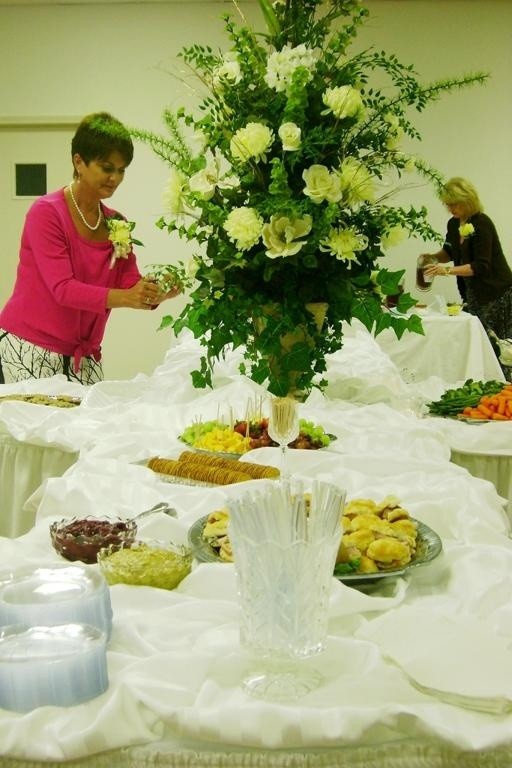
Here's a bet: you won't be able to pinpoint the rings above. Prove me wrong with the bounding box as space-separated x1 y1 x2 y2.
146 297 153 304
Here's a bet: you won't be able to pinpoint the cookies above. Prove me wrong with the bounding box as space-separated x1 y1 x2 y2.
147 451 280 483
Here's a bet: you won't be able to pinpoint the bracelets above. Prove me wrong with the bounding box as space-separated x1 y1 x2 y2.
444 265 452 278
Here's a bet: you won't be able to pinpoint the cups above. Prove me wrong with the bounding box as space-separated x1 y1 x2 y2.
386 278 406 308
223 476 349 702
415 255 439 292
446 297 463 316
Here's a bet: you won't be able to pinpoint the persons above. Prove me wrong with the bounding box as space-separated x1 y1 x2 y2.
417 177 512 388
1 113 186 388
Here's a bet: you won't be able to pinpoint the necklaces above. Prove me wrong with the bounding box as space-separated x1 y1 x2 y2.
69 179 102 231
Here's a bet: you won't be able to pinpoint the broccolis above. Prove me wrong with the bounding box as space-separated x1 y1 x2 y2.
448 378 502 397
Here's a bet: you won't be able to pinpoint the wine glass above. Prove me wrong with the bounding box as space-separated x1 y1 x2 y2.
267 397 300 470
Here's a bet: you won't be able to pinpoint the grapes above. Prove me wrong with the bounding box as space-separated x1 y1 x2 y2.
299 418 330 446
184 419 226 443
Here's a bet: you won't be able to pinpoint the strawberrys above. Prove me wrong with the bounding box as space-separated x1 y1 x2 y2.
233 419 273 449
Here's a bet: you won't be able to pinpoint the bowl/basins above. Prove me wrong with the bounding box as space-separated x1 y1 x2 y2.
50 513 192 590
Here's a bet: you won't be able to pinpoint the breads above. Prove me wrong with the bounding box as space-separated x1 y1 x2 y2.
202 492 419 573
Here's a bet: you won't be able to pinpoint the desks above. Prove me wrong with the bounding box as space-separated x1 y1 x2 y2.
0 307 512 768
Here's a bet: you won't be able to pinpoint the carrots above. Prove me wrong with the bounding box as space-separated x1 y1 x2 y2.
464 386 512 420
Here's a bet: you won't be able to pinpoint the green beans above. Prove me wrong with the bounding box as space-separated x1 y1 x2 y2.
426 396 481 417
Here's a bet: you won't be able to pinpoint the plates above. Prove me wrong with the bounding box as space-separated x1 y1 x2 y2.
178 433 339 461
188 507 443 588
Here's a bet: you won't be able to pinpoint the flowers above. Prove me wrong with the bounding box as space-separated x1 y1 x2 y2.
83 0 490 401
